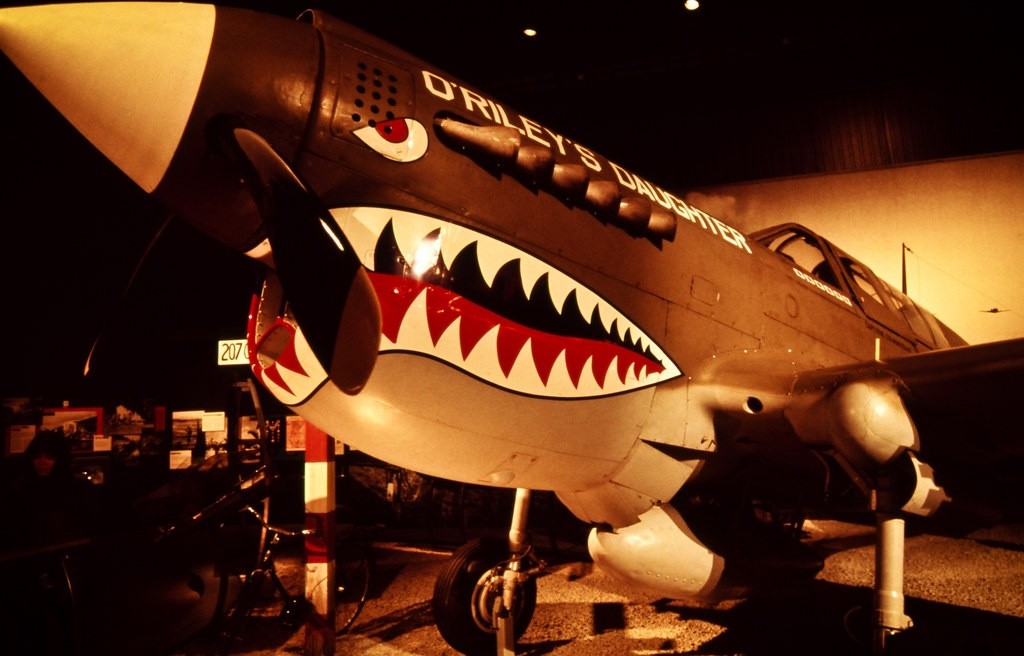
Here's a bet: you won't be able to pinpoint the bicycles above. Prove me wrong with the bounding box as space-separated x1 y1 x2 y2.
218 501 386 652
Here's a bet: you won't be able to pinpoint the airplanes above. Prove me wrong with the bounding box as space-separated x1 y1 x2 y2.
1 1 1023 655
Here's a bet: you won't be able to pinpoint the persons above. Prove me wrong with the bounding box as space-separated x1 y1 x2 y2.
8 430 96 654
